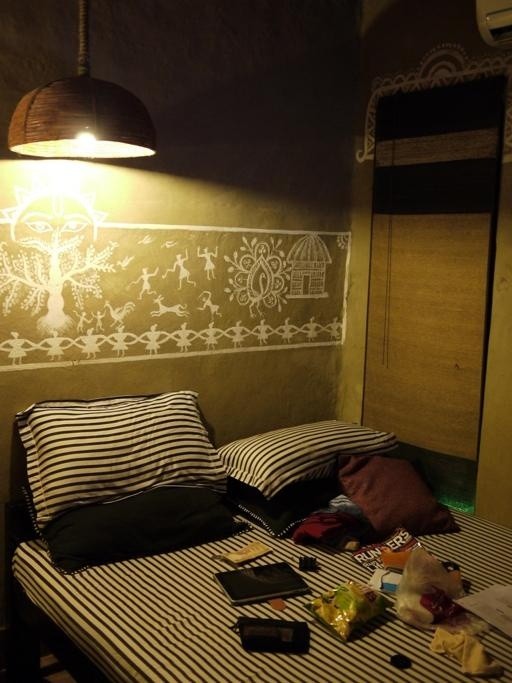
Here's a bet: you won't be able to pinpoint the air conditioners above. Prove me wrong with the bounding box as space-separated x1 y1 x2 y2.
475 0 512 49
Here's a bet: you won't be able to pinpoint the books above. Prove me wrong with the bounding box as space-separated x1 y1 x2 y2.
352 525 427 573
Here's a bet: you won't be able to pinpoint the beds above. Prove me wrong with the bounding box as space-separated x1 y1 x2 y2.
0 505 512 683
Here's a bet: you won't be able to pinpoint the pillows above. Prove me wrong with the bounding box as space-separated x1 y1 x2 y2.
341 455 460 538
231 454 395 539
14 390 253 535
18 472 251 576
218 419 400 501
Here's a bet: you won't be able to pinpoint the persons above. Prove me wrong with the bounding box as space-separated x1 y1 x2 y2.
379 544 393 554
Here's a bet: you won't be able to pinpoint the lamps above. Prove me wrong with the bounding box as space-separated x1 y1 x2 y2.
6 0 158 159
